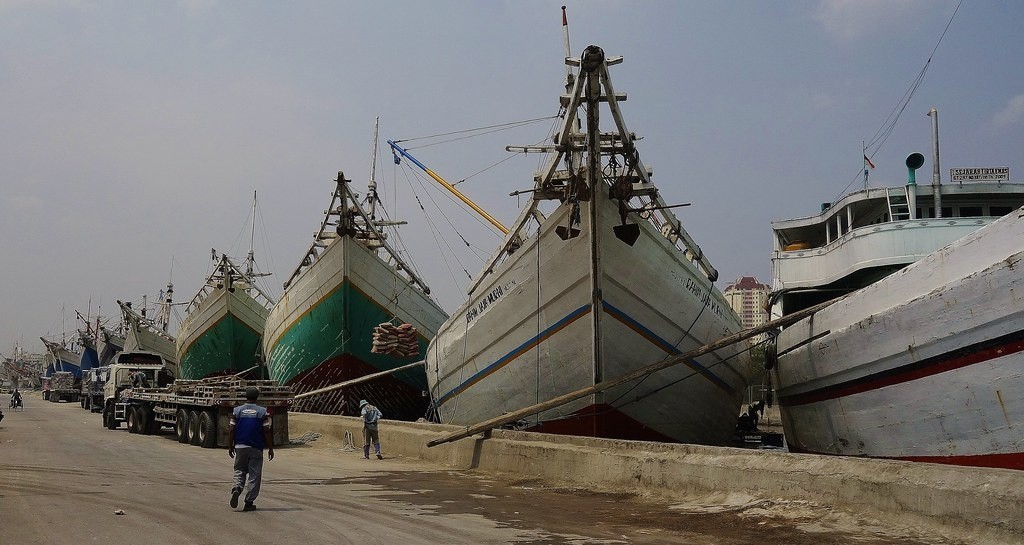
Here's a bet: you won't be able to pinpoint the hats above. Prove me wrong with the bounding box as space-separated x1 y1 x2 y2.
246 387 259 400
359 400 368 407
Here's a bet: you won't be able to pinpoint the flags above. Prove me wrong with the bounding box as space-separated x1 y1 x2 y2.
864 155 875 169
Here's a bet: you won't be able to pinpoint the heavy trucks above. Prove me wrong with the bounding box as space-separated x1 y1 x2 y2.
102 351 295 448
79 367 108 413
42 372 79 402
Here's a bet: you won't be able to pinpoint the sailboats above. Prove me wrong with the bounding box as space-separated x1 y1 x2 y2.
175 190 276 381
262 117 450 422
388 5 755 447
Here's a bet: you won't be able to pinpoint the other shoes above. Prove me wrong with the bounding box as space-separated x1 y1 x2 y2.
364 454 370 459
243 499 256 510
376 452 383 460
229 490 240 508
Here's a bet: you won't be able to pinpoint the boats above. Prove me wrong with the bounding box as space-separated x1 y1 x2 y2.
765 107 1024 471
39 256 177 377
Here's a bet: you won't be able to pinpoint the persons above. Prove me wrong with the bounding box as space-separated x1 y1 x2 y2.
228 387 274 512
359 400 382 460
11 388 22 408
128 370 151 392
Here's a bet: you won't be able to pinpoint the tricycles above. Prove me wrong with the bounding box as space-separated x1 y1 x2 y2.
9 397 23 412
